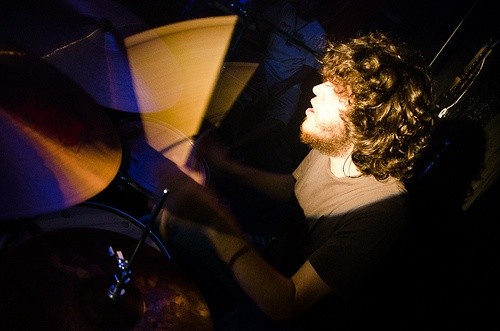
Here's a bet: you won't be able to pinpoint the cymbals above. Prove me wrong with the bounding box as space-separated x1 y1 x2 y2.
0 49 123 220
50 257 212 331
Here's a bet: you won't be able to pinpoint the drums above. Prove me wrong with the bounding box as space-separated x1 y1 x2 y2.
117 115 211 211
0 203 176 330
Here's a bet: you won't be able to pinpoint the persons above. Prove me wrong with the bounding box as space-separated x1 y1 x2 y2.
151 34 433 330
243 0 328 145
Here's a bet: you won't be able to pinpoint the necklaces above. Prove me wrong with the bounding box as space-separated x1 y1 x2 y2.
293 10 314 33
